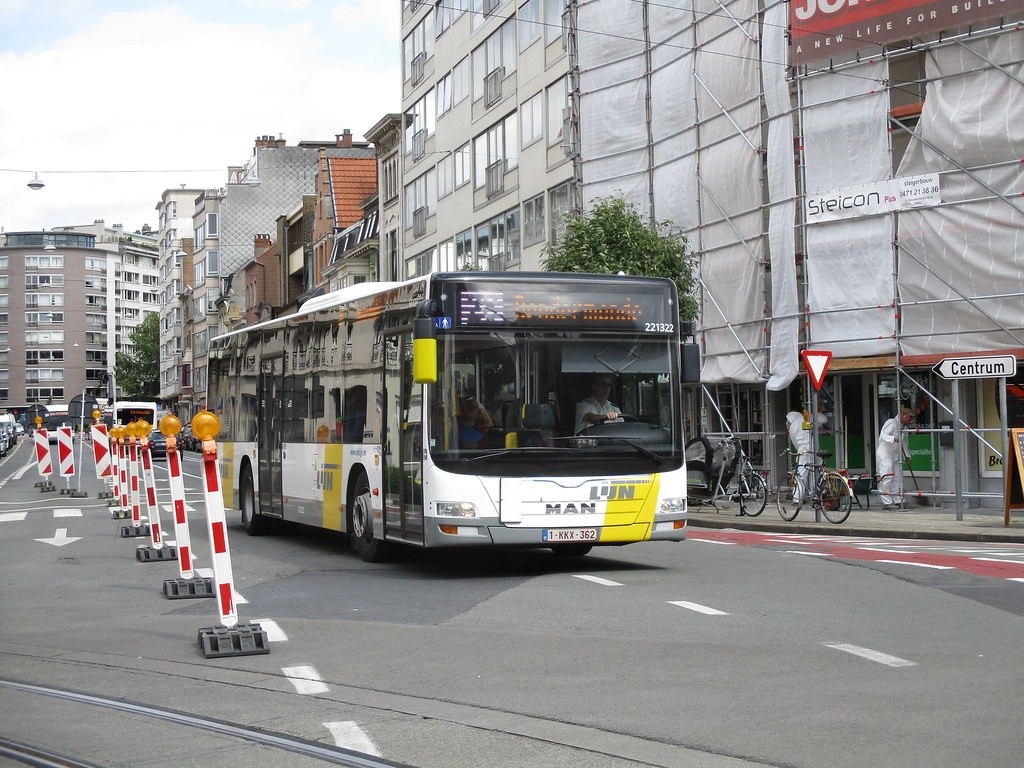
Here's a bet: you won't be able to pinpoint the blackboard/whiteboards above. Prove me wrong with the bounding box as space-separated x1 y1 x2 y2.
1005 428 1024 509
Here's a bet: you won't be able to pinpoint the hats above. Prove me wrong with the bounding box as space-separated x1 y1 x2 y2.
900 407 916 417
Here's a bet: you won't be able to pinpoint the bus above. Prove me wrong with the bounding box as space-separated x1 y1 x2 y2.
112 401 158 454
204 272 700 564
44 412 75 444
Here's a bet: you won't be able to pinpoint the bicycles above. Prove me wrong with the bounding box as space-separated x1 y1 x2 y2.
777 447 853 524
722 431 771 517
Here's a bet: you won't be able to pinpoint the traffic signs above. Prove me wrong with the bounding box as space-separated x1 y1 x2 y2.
931 353 1018 380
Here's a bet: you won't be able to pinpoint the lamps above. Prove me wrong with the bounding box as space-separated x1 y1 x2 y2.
73 341 80 347
46 311 54 318
43 241 56 253
150 284 158 293
26 171 46 191
5 346 12 352
125 311 132 318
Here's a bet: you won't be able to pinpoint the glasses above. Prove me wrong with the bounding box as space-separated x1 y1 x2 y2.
466 404 479 409
593 382 614 388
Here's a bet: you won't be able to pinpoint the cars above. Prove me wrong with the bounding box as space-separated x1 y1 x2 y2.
28 424 48 437
179 422 202 453
148 429 183 462
0 413 26 459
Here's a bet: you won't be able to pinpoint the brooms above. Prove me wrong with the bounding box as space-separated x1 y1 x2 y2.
897 440 929 506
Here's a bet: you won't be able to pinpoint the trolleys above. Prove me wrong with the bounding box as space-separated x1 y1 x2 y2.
827 472 895 509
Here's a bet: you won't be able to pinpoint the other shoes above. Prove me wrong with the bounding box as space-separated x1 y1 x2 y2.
883 504 900 510
896 501 914 509
790 502 799 506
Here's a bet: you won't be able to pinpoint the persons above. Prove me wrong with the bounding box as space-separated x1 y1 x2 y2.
789 404 829 505
455 369 513 448
875 410 911 510
574 374 625 446
